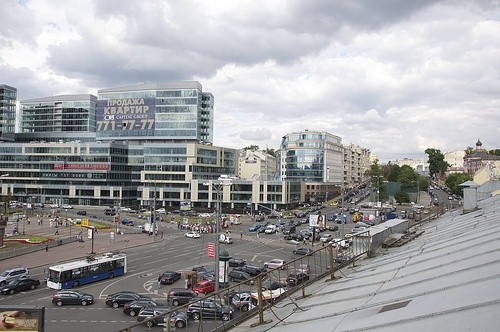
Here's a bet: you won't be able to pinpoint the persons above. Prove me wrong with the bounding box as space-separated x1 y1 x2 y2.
38 219 40 225
177 220 181 229
27 218 30 224
321 241 325 248
95 227 97 232
256 230 259 238
40 218 43 224
157 274 161 288
56 229 58 233
185 277 189 289
317 215 323 225
45 243 48 252
303 237 307 245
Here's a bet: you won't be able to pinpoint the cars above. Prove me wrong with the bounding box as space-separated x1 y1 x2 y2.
187 302 234 321
262 282 286 291
158 256 310 306
231 293 250 312
224 291 251 304
52 291 94 307
106 291 151 308
123 300 166 317
249 288 287 311
137 306 188 329
8 182 463 263
2 278 40 295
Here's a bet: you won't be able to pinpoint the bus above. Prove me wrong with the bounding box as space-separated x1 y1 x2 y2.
0 267 29 286
46 251 127 290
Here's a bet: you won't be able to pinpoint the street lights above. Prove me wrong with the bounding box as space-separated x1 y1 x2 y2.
411 181 420 204
141 179 157 240
372 176 384 205
335 184 354 238
204 181 234 291
